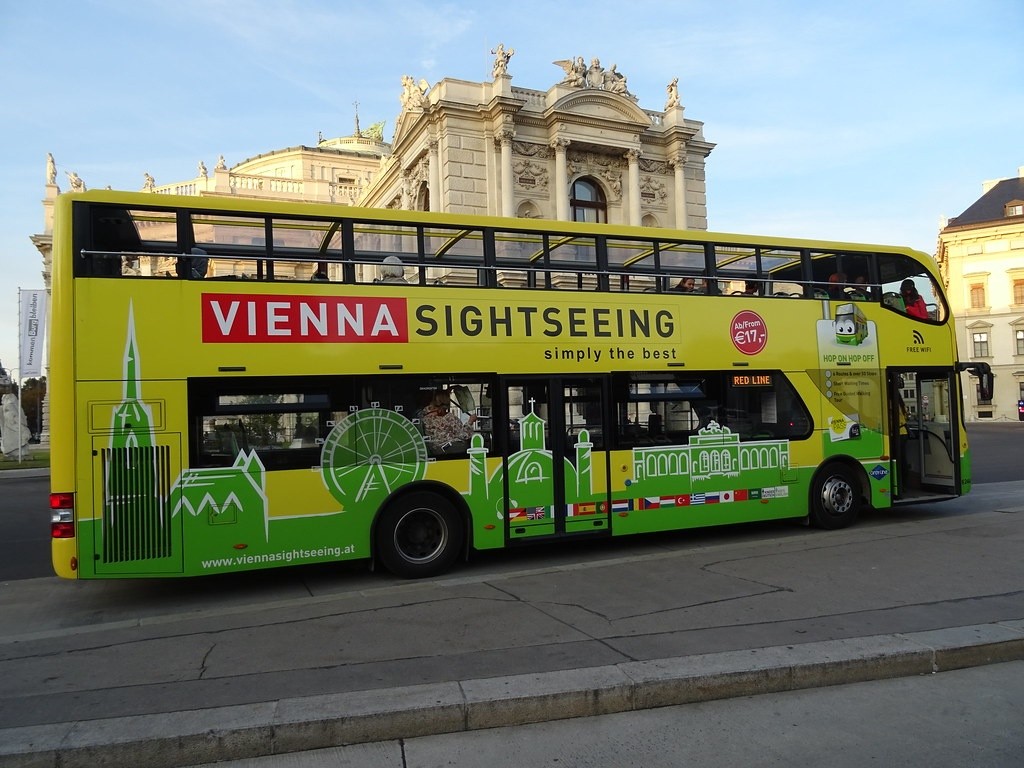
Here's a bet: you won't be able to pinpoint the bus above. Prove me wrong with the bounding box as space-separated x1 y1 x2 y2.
48 190 994 581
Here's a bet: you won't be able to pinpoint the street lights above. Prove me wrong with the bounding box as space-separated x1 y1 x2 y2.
1 368 19 394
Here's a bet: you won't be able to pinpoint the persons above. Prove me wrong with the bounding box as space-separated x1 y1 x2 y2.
174 246 211 281
742 274 762 298
375 254 408 285
215 155 227 169
491 42 514 72
143 171 156 189
400 74 429 108
668 267 724 295
824 271 880 303
197 159 209 177
46 152 58 183
898 279 930 322
666 79 682 107
415 388 477 457
566 54 634 97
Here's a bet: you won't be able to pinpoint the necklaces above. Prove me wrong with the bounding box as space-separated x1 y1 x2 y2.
895 393 916 493
